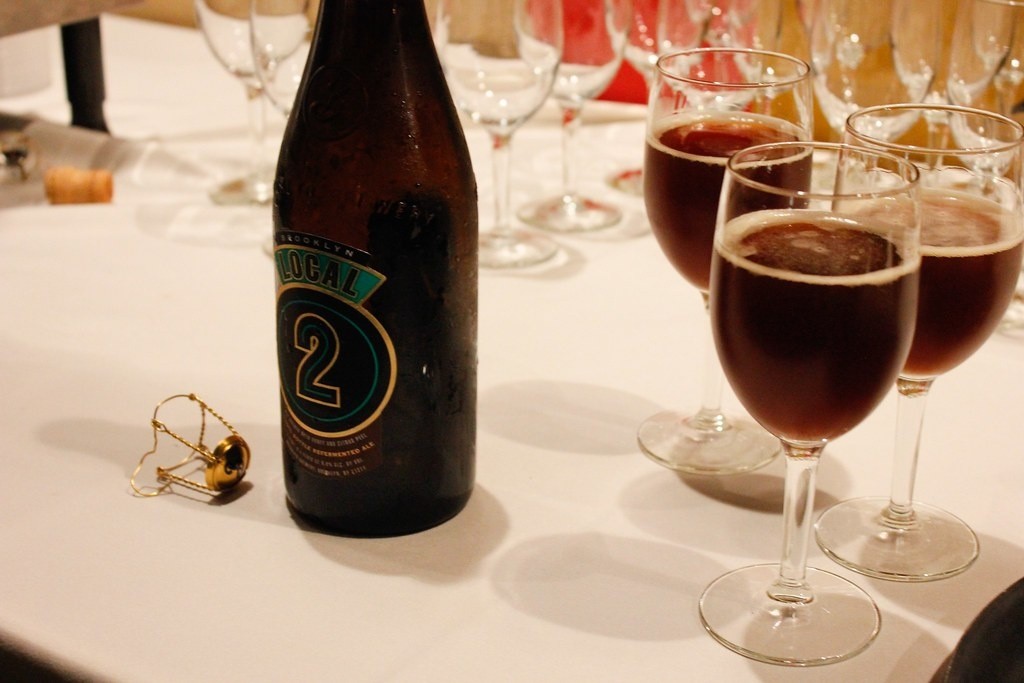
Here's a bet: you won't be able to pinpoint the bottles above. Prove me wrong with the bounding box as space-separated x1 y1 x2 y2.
274 1 478 538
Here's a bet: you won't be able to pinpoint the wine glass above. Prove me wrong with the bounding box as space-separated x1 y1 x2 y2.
698 142 919 666
814 104 1017 585
196 1 319 209
639 49 812 476
424 1 1024 332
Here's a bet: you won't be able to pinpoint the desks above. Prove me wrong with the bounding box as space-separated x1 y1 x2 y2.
0 13 1024 683
